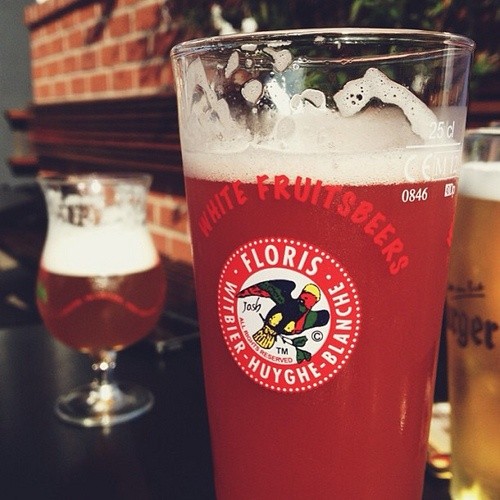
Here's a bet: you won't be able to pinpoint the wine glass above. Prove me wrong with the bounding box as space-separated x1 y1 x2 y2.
35 173 168 427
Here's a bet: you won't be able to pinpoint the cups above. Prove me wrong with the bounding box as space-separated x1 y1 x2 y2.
171 28 476 500
445 128 500 500
